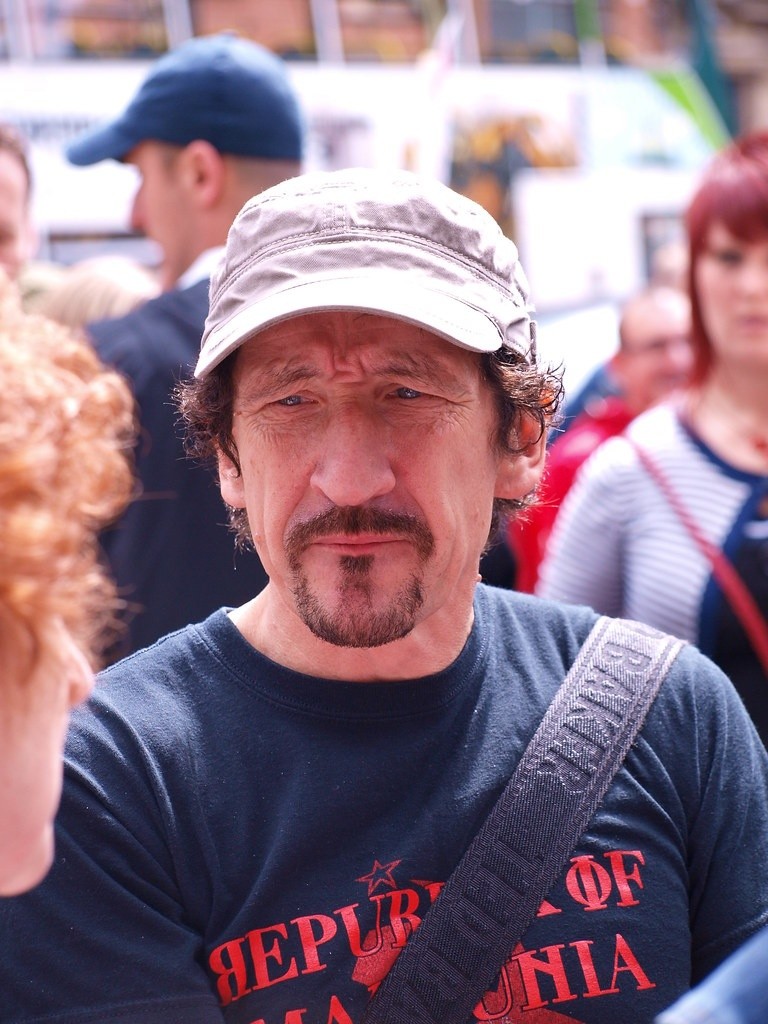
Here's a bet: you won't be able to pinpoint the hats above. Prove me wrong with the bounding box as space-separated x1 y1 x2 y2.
64 33 303 169
191 168 532 382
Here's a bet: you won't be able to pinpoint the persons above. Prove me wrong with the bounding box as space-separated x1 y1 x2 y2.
1 28 306 665
506 132 767 741
0 279 137 896
0 164 768 1024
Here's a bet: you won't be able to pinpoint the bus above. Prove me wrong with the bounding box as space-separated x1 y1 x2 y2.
0 56 716 403
